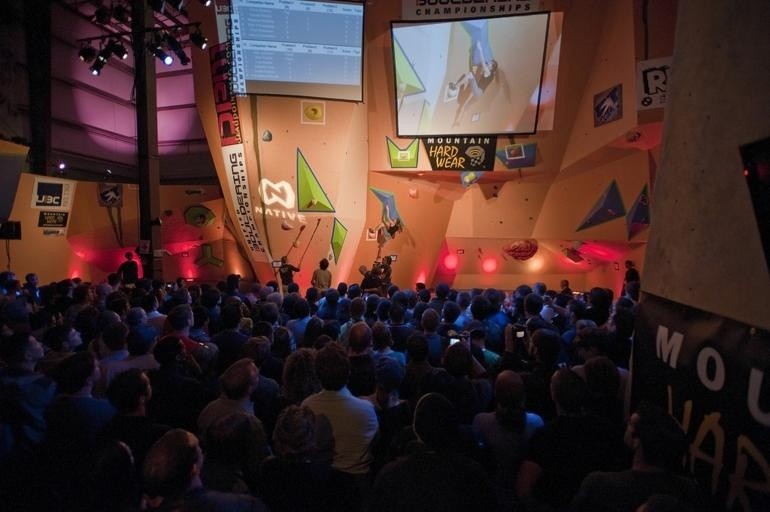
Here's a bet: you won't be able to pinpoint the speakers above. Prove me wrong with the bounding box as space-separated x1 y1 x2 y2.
0 221 21 240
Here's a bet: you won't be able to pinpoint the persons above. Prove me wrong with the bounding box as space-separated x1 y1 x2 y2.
1 273 701 511
278 256 302 296
354 261 382 289
117 251 140 290
311 258 333 291
373 256 393 285
621 260 640 293
449 41 498 126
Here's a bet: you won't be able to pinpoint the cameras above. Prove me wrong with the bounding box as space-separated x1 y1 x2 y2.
513 323 527 341
448 334 469 346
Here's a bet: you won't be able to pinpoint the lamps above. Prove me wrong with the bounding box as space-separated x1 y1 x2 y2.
75 0 214 76
